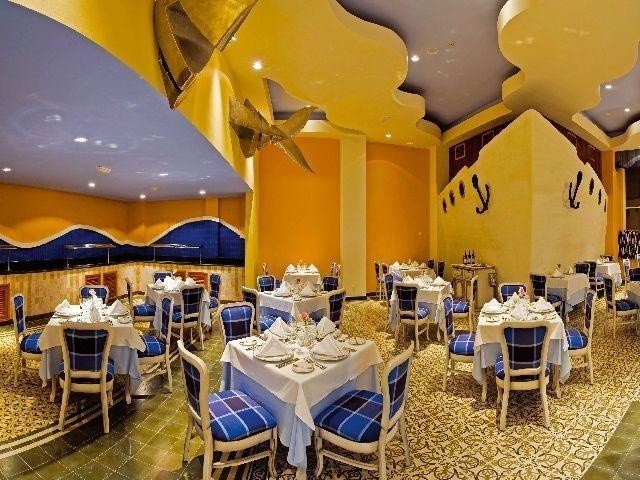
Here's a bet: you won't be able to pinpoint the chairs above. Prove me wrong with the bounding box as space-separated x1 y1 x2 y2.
153 273 173 285
12 293 48 387
59 325 115 434
125 279 155 328
78 284 109 308
138 296 174 391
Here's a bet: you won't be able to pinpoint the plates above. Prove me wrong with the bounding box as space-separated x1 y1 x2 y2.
387 261 451 293
241 321 365 376
481 300 557 325
52 301 130 329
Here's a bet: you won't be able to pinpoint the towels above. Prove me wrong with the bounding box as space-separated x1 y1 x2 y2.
82 306 102 320
107 297 129 314
55 298 76 314
